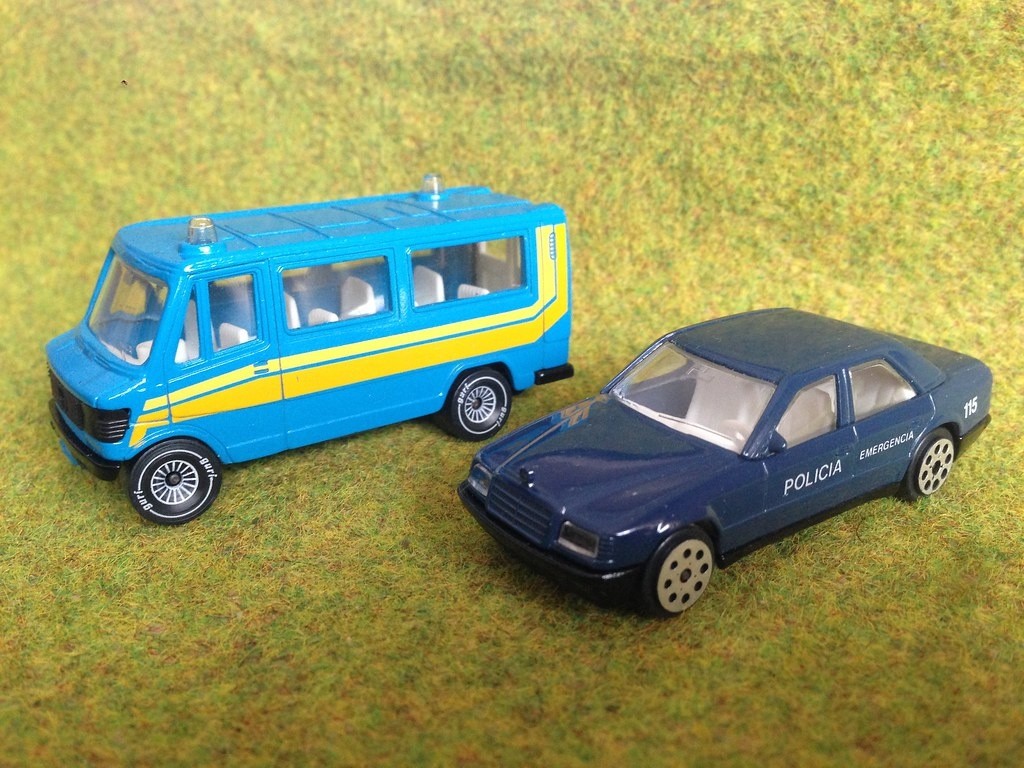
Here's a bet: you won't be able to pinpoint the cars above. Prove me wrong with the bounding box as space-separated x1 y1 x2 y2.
458 307 994 615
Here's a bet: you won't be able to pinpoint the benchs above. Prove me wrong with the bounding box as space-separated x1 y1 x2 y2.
851 366 916 416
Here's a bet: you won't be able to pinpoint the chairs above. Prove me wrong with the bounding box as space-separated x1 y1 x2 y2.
685 366 835 447
185 264 489 360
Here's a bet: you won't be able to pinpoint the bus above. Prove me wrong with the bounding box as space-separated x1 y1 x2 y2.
43 173 579 526
